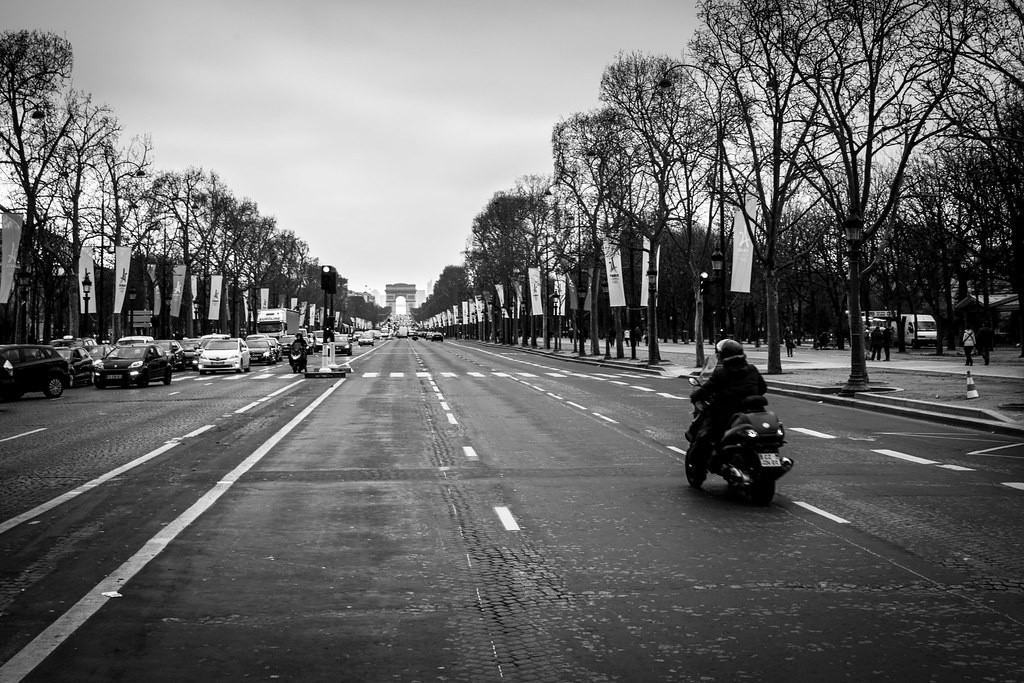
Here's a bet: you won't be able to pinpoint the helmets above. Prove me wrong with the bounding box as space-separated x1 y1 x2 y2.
716 339 743 361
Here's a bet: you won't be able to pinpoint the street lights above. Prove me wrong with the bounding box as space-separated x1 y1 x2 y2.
472 313 475 338
82 268 92 336
508 302 514 345
192 295 200 338
501 304 506 345
551 291 560 352
646 265 659 365
493 307 498 344
520 298 528 346
17 263 32 344
481 310 484 341
601 280 612 360
128 285 137 336
577 286 587 357
709 241 724 355
467 314 469 340
164 294 173 340
839 204 870 397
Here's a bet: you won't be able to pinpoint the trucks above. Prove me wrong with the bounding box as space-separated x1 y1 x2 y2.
399 326 408 339
892 314 937 348
253 308 300 338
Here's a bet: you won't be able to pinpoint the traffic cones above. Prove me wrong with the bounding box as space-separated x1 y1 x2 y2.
966 371 979 399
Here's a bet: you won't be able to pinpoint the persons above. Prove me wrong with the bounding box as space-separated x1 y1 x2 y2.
643 327 649 346
624 327 630 347
783 329 794 357
865 326 892 361
292 334 307 372
635 327 641 346
819 333 830 347
977 321 994 365
569 331 580 343
690 338 767 482
609 327 616 346
962 325 976 366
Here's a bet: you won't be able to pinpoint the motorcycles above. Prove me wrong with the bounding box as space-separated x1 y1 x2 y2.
685 377 793 507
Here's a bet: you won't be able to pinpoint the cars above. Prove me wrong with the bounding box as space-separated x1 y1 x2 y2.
0 329 352 404
348 317 399 346
408 326 446 342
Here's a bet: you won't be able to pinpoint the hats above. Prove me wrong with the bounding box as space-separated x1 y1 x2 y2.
295 333 302 337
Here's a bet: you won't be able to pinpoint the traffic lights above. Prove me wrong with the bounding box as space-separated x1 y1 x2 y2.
700 272 710 295
322 265 332 281
